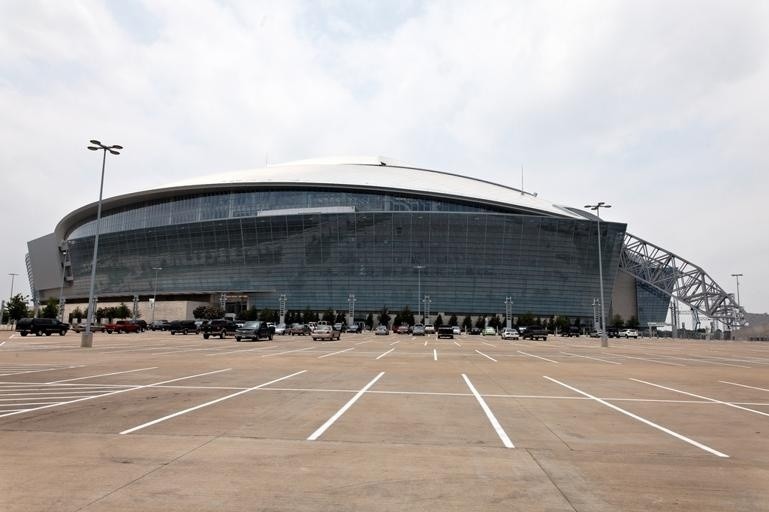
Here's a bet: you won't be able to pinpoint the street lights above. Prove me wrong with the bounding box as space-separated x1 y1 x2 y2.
57 239 77 319
584 201 612 347
730 273 744 307
412 264 427 324
8 273 20 300
80 139 124 346
151 267 162 323
